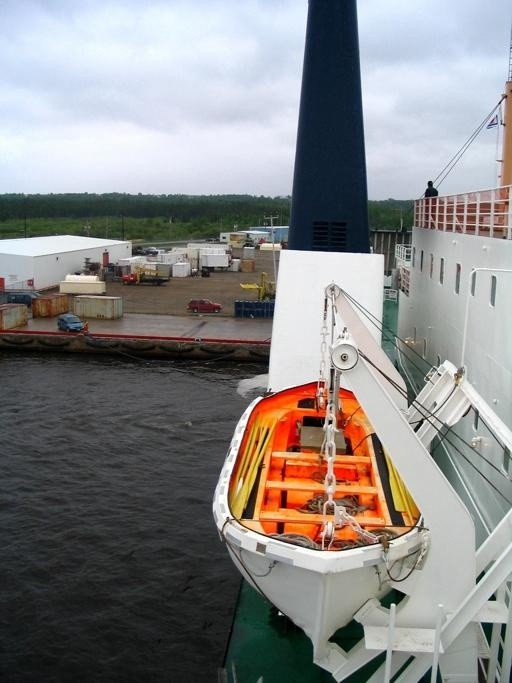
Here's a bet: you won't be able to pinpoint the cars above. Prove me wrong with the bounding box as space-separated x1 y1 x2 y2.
136 246 160 255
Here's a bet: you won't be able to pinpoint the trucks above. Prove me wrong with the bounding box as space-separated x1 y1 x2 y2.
122 267 170 285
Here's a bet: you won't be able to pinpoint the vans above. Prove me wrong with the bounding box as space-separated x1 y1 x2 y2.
58 313 86 332
7 292 40 307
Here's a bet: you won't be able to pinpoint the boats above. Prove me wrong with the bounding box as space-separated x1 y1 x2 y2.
212 373 428 664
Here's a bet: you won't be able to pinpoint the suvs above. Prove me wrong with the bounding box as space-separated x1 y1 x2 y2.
186 296 222 313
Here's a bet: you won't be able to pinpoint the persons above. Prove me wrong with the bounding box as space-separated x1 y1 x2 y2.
424 181 438 229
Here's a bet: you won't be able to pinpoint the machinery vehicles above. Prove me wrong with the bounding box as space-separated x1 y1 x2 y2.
240 271 276 303
